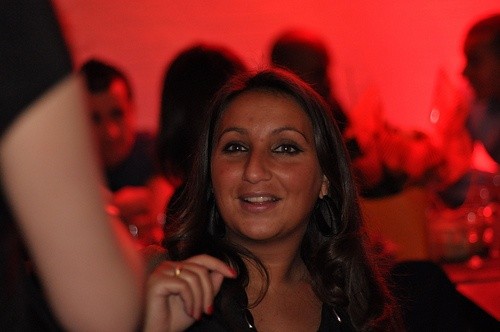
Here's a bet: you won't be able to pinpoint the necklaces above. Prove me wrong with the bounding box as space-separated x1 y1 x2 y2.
244 308 343 332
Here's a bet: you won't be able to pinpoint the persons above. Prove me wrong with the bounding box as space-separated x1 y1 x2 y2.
77 13 500 220
148 68 500 332
0 0 144 332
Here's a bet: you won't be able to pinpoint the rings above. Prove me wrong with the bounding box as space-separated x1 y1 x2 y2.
174 265 183 278
